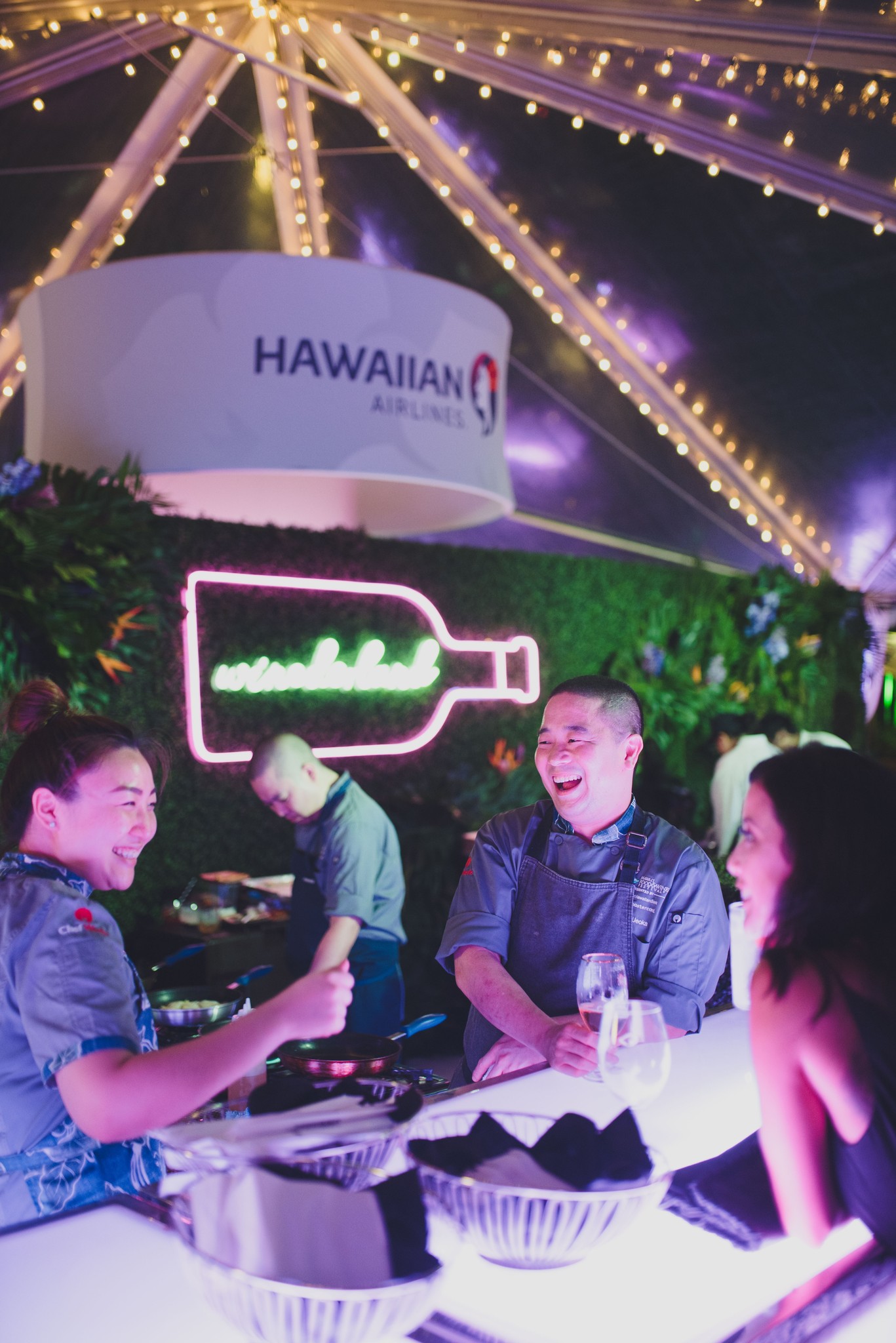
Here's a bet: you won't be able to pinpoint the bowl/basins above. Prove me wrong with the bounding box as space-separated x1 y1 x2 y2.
396 1110 674 1268
157 1079 426 1192
167 1159 456 1343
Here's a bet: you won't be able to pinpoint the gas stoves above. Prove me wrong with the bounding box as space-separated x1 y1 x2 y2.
157 1025 456 1119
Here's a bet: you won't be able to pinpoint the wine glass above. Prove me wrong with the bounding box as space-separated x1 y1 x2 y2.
576 951 629 1084
596 999 670 1146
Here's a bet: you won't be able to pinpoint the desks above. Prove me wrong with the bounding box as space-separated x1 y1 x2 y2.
0 1000 870 1342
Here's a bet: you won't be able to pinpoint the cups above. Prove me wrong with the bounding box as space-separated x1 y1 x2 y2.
728 901 763 1012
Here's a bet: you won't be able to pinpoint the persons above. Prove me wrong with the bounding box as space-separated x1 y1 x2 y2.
758 710 852 749
726 740 896 1242
248 732 406 1056
710 711 782 857
0 711 351 1231
434 675 729 1083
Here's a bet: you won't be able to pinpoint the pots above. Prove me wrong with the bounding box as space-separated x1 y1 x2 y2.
131 943 208 990
274 1013 447 1080
145 965 275 1027
